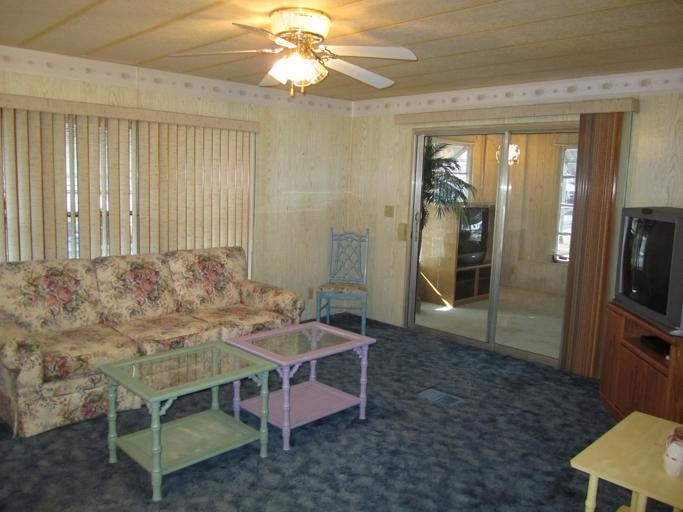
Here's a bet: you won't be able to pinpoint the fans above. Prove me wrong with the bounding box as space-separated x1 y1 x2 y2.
166 8 417 91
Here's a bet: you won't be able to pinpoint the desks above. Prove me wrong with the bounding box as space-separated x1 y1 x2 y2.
569 410 682 511
102 339 278 500
219 320 378 450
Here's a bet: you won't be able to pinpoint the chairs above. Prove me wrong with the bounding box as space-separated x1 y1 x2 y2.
316 226 370 336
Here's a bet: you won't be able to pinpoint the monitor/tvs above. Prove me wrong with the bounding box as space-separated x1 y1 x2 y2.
611 206 683 336
458 205 489 266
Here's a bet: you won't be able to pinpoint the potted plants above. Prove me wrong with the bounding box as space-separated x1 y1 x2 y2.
416 135 480 314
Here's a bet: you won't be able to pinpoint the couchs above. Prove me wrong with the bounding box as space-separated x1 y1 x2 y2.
2 247 305 439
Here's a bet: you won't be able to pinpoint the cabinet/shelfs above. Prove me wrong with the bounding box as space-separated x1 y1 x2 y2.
418 200 496 307
597 300 683 422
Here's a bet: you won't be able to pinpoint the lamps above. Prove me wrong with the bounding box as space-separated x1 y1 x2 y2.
268 45 329 88
